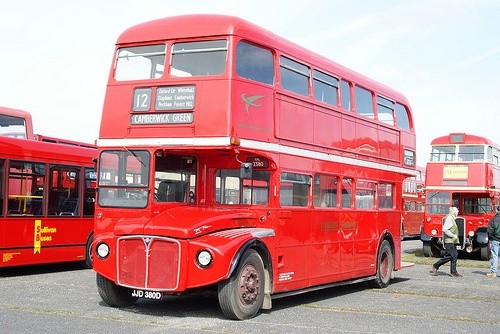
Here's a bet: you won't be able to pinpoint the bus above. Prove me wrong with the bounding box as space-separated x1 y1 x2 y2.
420 132 500 260
377 166 426 241
91 13 417 321
0 106 142 215
0 136 141 268
244 179 292 207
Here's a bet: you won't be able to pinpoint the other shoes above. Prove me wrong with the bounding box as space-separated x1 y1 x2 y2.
487 273 497 278
431 264 438 276
450 272 464 277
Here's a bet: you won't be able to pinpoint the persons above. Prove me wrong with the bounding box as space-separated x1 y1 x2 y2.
486 206 500 277
431 207 464 278
